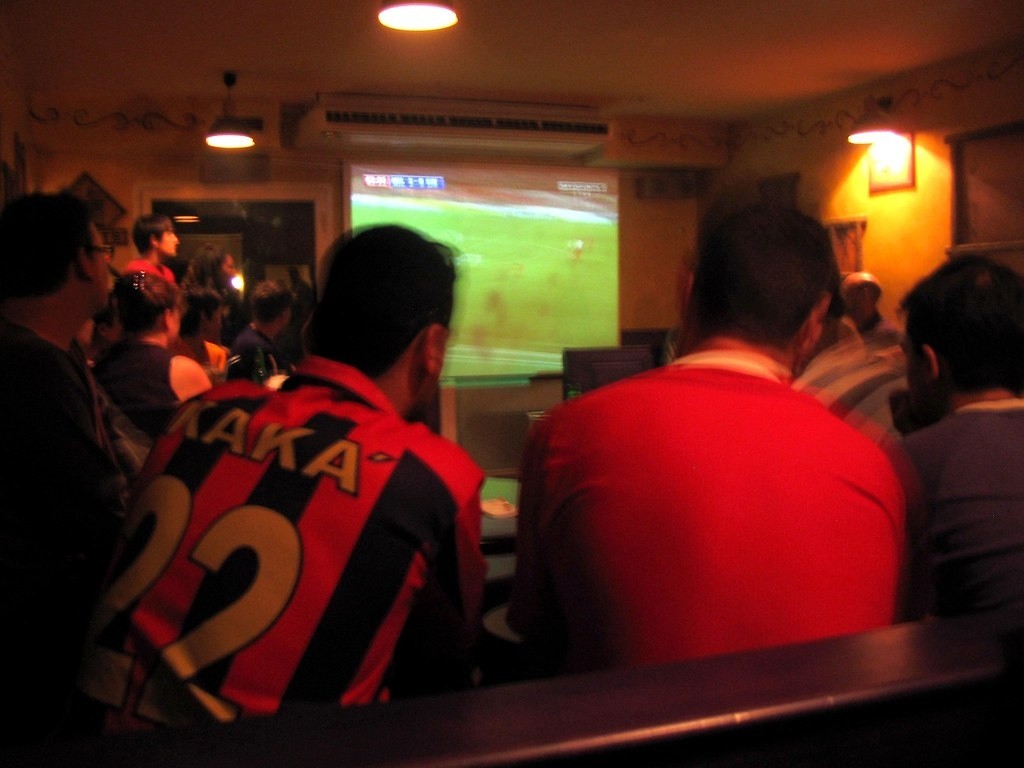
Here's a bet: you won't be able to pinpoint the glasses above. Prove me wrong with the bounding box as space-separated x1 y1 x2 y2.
89 244 114 263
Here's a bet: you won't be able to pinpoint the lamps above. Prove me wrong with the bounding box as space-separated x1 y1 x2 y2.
203 73 262 149
847 89 895 146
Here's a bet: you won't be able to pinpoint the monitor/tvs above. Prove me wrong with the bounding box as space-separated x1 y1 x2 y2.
561 344 655 402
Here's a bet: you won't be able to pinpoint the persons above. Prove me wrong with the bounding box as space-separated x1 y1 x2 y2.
794 254 1024 623
0 190 132 715
507 202 904 678
102 224 487 730
76 214 306 713
